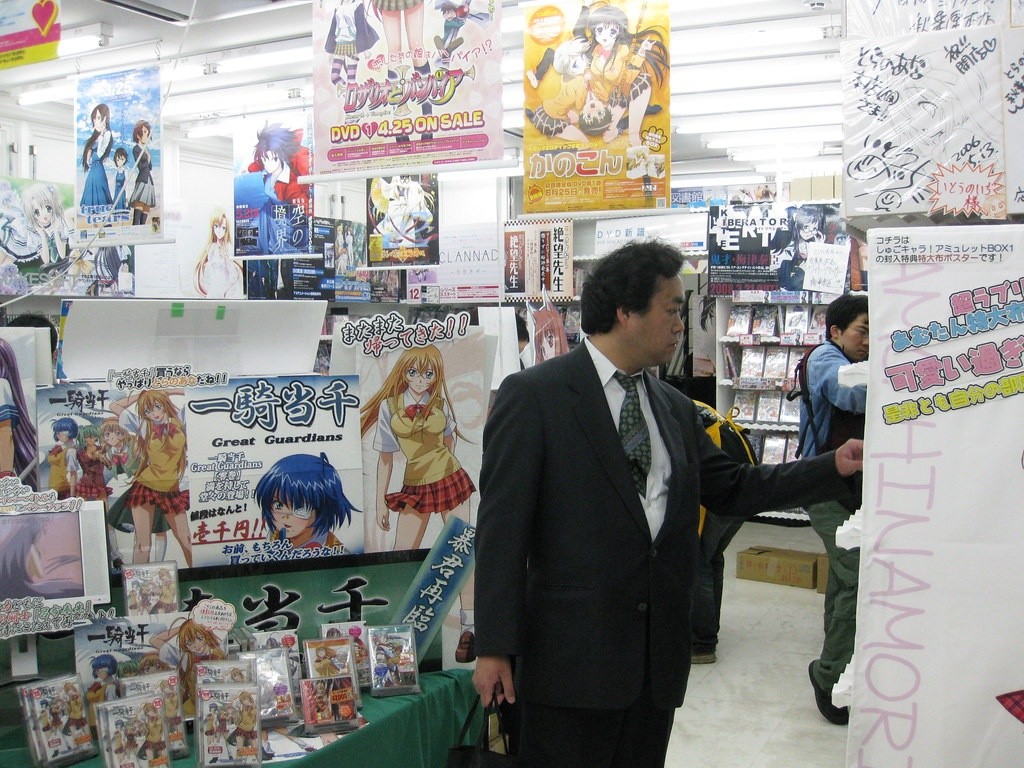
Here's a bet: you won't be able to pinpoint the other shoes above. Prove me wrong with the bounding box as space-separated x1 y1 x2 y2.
690 642 717 663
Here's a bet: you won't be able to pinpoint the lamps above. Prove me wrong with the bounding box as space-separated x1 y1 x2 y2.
0 0 313 109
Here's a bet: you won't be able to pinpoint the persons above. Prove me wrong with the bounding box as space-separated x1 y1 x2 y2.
6 312 58 386
472 238 863 768
691 399 759 664
800 294 869 724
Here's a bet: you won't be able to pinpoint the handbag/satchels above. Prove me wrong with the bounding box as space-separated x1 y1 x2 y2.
446 692 516 768
786 343 866 514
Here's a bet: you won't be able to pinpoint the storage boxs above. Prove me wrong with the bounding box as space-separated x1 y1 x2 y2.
723 290 842 466
735 546 822 590
818 552 831 595
0 558 425 768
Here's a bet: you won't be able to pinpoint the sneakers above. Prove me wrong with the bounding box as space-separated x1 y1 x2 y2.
809 660 850 725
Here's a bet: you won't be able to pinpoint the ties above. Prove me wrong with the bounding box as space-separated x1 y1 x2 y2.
613 371 652 500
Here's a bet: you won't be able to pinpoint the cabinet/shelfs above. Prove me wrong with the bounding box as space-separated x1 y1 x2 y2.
714 288 843 529
